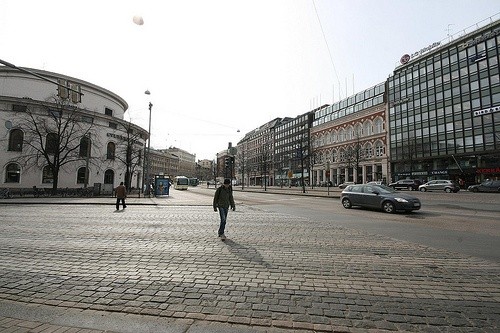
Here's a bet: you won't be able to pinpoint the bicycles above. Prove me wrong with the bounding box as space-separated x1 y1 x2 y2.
34 186 93 199
0 189 15 199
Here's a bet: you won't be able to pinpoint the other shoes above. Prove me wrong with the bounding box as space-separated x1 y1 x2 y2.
220 233 226 239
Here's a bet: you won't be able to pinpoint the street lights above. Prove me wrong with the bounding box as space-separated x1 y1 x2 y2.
83 132 91 196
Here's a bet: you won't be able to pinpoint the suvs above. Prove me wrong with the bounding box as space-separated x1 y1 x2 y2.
388 179 420 191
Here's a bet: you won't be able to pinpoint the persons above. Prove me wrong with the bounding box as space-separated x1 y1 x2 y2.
115 182 127 210
459 178 465 189
207 182 210 188
213 179 236 240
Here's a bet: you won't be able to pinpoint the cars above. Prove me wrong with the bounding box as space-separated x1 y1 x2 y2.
341 184 421 214
418 180 460 193
468 177 500 193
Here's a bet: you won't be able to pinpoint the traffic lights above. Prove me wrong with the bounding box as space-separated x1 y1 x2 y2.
323 162 325 170
225 157 231 169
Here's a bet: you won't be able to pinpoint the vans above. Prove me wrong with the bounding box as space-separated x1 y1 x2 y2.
339 181 385 189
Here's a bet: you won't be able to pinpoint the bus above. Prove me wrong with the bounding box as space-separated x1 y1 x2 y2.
155 174 169 197
173 176 188 190
187 178 197 187
